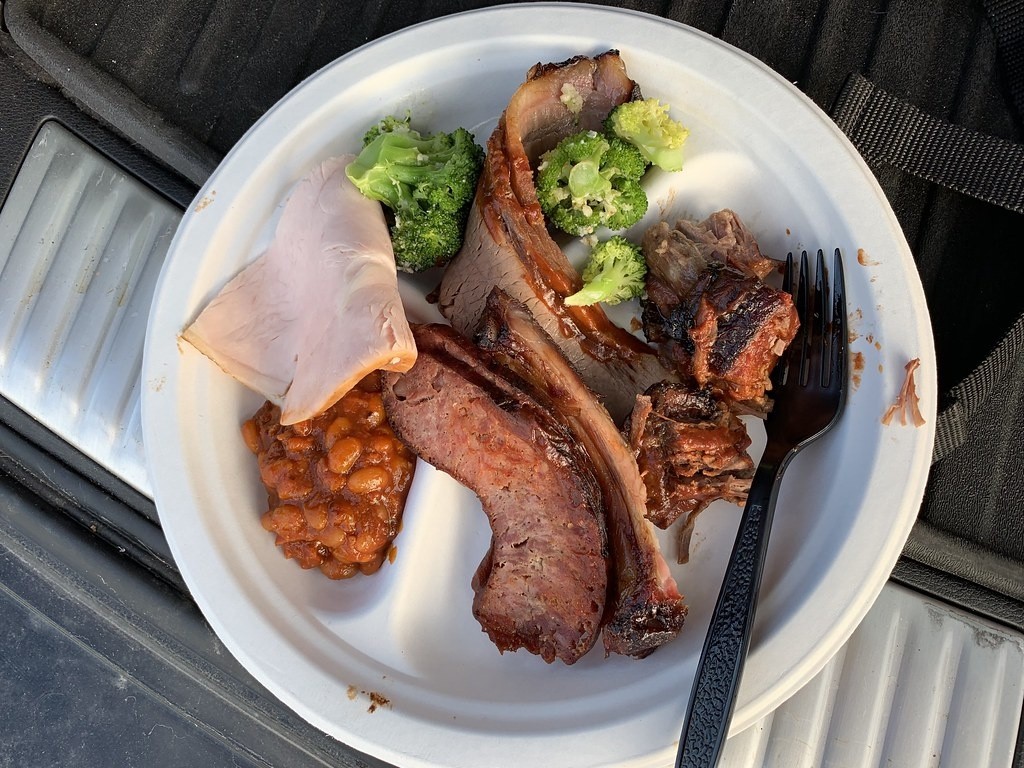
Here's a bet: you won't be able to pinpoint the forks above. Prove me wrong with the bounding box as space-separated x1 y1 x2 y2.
674 246 849 768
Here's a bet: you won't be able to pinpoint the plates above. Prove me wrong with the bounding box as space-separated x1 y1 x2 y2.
140 2 939 768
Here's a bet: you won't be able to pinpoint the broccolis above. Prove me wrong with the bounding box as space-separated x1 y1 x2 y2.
344 96 691 307
